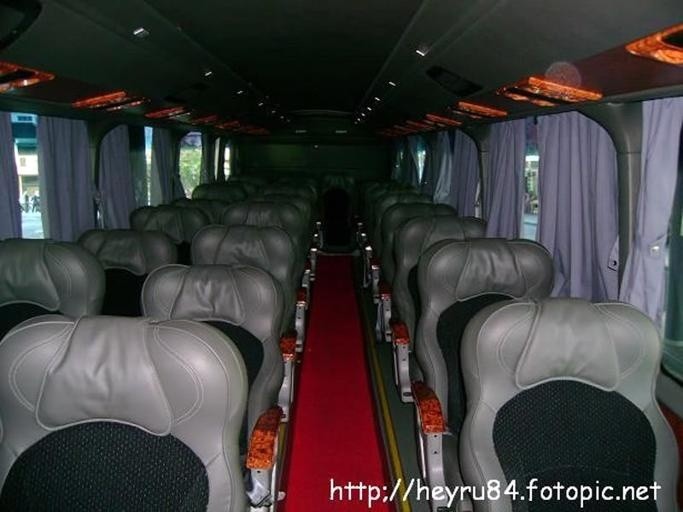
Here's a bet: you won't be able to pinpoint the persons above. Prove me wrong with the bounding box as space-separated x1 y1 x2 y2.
31 194 40 213
25 190 30 213
